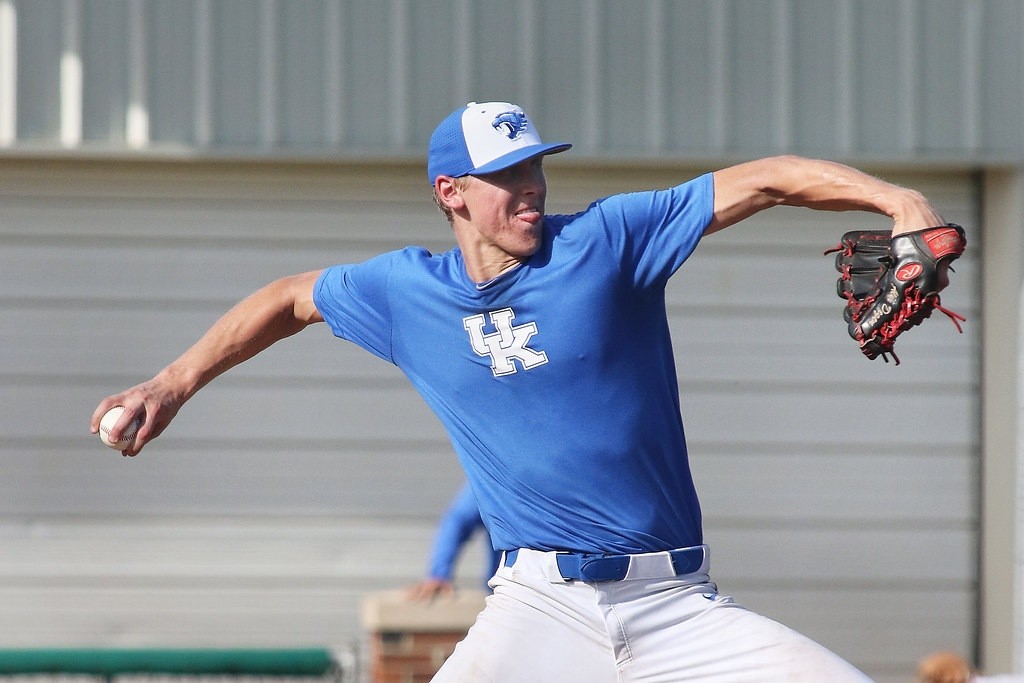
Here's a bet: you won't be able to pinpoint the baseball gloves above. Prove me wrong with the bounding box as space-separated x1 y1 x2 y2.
822 222 969 369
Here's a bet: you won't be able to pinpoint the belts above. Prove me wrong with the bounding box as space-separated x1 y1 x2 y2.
504 546 703 583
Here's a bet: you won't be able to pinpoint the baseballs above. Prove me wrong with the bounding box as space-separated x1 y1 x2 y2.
96 406 140 451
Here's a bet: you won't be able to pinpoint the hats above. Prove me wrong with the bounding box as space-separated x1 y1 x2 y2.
428 101 573 187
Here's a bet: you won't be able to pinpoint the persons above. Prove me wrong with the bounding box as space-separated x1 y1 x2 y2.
402 481 503 606
91 100 968 683
